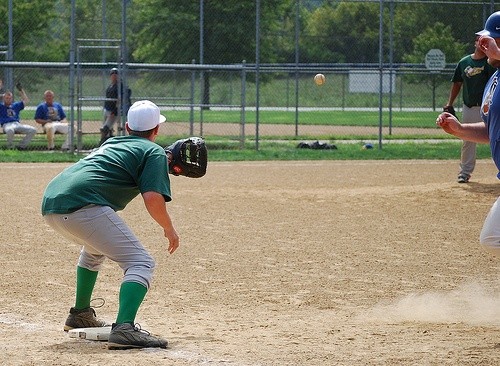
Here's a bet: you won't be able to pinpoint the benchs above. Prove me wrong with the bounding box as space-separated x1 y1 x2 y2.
0 131 102 134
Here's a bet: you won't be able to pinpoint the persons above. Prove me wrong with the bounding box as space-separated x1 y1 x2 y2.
41 100 179 349
99 69 122 148
0 79 36 149
34 90 77 151
436 11 500 256
442 35 497 183
0 79 6 133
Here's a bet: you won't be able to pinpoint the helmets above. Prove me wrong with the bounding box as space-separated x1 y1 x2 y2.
475 10 500 37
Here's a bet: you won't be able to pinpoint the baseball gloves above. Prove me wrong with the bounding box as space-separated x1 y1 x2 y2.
163 136 207 178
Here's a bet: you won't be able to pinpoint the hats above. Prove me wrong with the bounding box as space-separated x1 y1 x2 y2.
128 100 165 132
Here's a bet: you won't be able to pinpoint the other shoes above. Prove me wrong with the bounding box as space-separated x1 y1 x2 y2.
458 176 466 182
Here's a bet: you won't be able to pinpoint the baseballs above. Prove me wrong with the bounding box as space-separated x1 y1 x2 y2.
314 74 325 85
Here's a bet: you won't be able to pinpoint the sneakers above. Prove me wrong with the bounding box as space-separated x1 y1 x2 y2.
64 298 112 331
107 322 168 349
